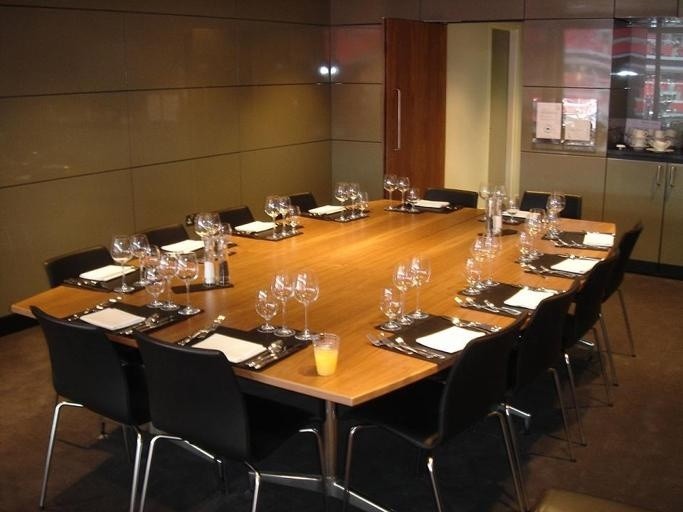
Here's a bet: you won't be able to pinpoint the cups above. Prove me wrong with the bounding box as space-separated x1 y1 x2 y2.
312 333 342 377
629 129 676 154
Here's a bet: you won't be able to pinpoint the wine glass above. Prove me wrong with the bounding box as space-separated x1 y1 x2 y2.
263 195 301 240
383 176 424 214
255 272 322 341
108 208 231 317
461 181 567 298
378 254 434 331
334 175 371 223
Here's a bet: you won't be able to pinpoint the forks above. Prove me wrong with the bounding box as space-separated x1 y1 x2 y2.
521 261 578 279
558 252 605 261
454 295 523 317
442 312 503 334
582 229 616 237
177 314 226 347
365 331 447 360
549 236 609 252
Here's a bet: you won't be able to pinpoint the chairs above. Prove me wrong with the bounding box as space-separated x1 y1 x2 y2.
43 245 113 288
289 191 318 212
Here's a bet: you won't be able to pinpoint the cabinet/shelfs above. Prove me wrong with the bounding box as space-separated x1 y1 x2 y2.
603 157 682 266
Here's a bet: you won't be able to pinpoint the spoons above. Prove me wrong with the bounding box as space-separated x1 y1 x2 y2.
243 339 307 369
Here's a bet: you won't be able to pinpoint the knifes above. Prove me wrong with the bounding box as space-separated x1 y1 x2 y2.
117 303 177 336
65 298 118 322
